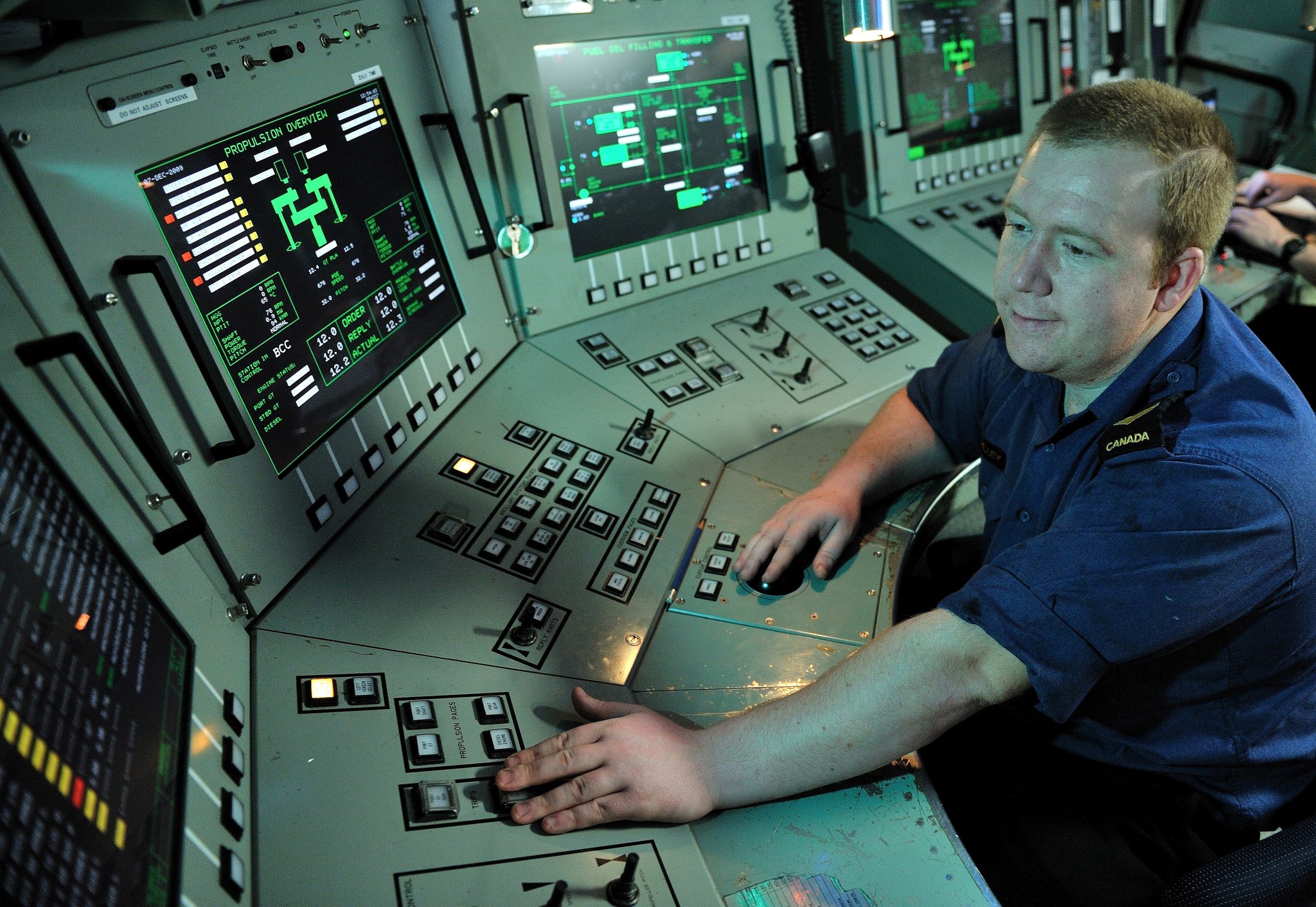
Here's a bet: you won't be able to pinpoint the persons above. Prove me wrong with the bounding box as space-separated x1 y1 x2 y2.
1229 167 1316 289
491 83 1316 907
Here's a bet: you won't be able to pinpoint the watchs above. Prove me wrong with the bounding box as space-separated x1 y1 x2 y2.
1281 238 1307 268
